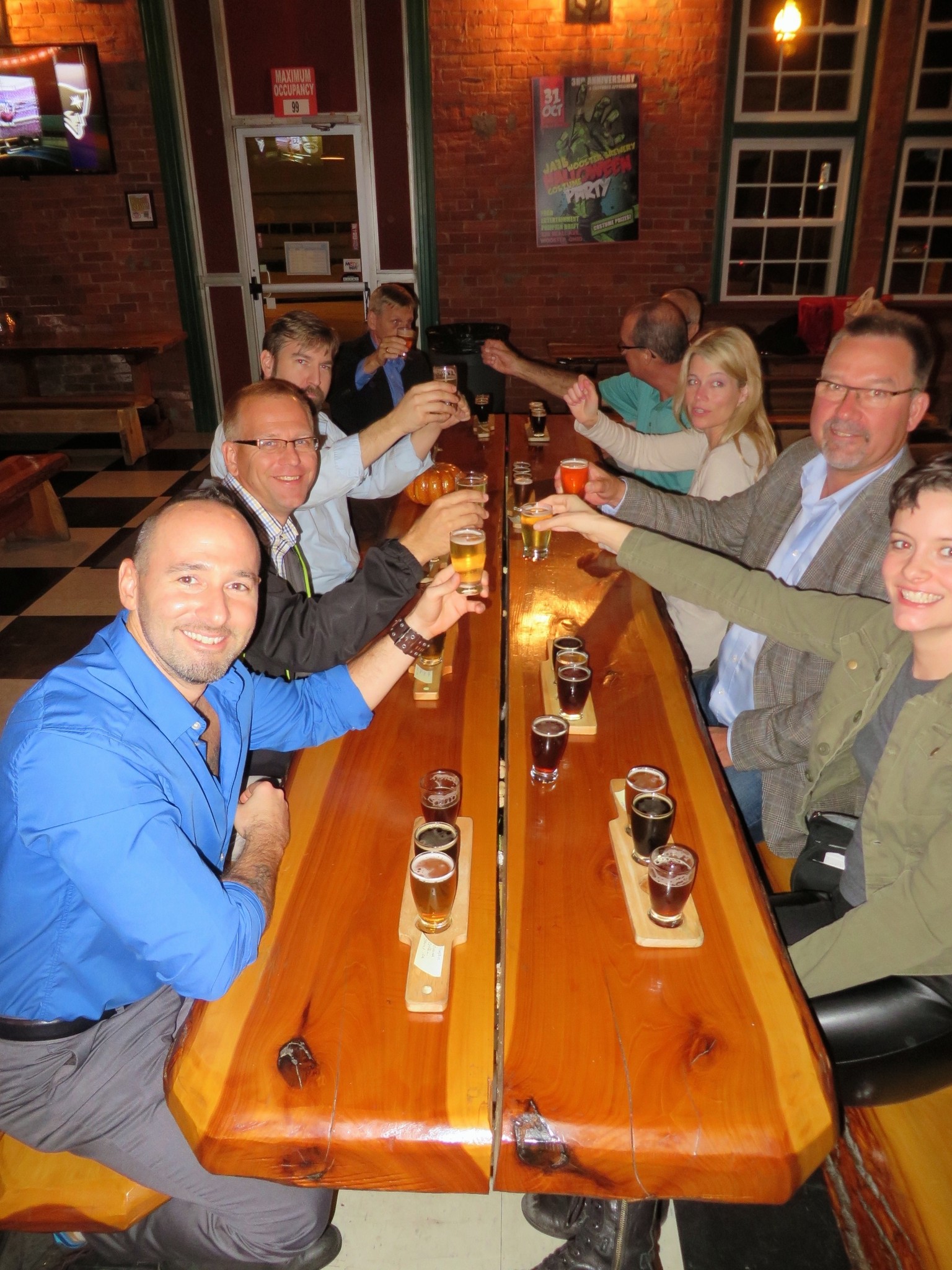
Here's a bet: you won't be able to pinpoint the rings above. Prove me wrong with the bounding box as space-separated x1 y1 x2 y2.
386 347 388 354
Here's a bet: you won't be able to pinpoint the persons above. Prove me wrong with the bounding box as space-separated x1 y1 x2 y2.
0 477 491 1268
481 298 700 495
659 287 702 348
553 313 938 858
191 378 490 868
508 444 952 1269
209 312 473 603
563 325 782 673
324 285 443 461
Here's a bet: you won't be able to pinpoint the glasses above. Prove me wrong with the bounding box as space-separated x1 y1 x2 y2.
231 436 319 453
814 376 925 407
617 340 656 359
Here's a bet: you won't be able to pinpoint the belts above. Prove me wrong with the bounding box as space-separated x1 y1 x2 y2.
0 1002 134 1042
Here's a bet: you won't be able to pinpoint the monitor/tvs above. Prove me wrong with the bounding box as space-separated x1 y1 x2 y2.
0 42 118 177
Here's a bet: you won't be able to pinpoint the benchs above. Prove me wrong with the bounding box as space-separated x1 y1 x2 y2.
747 833 952 1270
0 453 72 541
1 1131 174 1233
0 348 156 466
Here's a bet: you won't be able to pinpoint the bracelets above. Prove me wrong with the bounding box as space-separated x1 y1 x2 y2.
387 617 434 661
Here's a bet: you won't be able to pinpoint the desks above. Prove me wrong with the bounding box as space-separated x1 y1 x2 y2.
546 340 627 378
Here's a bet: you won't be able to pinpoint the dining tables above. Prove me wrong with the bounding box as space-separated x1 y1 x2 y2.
159 393 843 1215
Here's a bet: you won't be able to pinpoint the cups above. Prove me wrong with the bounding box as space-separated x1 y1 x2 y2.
381 325 716 938
